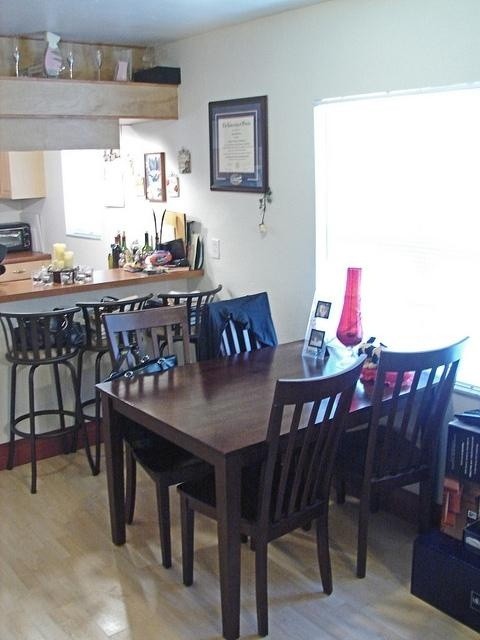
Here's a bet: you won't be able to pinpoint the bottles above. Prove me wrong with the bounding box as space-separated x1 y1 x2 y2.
107 231 153 269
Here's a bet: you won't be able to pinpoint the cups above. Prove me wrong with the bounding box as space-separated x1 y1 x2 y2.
32 264 94 286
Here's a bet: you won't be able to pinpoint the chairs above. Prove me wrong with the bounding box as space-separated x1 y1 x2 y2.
75 293 152 471
177 351 367 637
147 284 221 360
0 306 95 494
199 292 279 356
101 303 198 570
333 336 469 578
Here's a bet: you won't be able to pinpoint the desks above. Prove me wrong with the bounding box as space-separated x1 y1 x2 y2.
95 337 441 640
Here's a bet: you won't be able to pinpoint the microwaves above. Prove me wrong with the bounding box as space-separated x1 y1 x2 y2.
0 222 31 253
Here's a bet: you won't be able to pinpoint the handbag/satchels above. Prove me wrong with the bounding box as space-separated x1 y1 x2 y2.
12 290 204 351
103 354 195 473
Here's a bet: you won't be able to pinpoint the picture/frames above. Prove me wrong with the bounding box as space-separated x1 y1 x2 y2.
144 152 166 202
208 95 269 195
